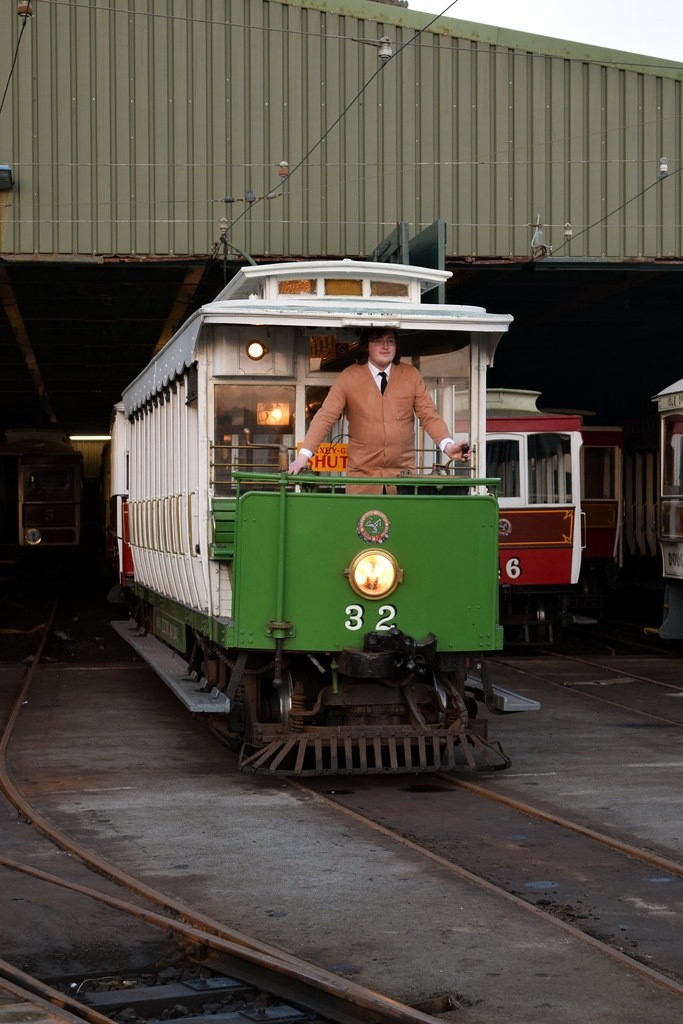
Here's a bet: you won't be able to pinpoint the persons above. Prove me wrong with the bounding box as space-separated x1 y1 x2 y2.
283 327 473 496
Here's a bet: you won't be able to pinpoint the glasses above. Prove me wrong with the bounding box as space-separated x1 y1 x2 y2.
368 339 396 346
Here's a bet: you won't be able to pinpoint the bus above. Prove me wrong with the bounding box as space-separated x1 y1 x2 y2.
2 427 81 565
96 199 545 785
640 378 683 650
438 385 589 651
543 409 626 631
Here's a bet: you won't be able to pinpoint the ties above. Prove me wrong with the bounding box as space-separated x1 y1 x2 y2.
377 372 388 396
